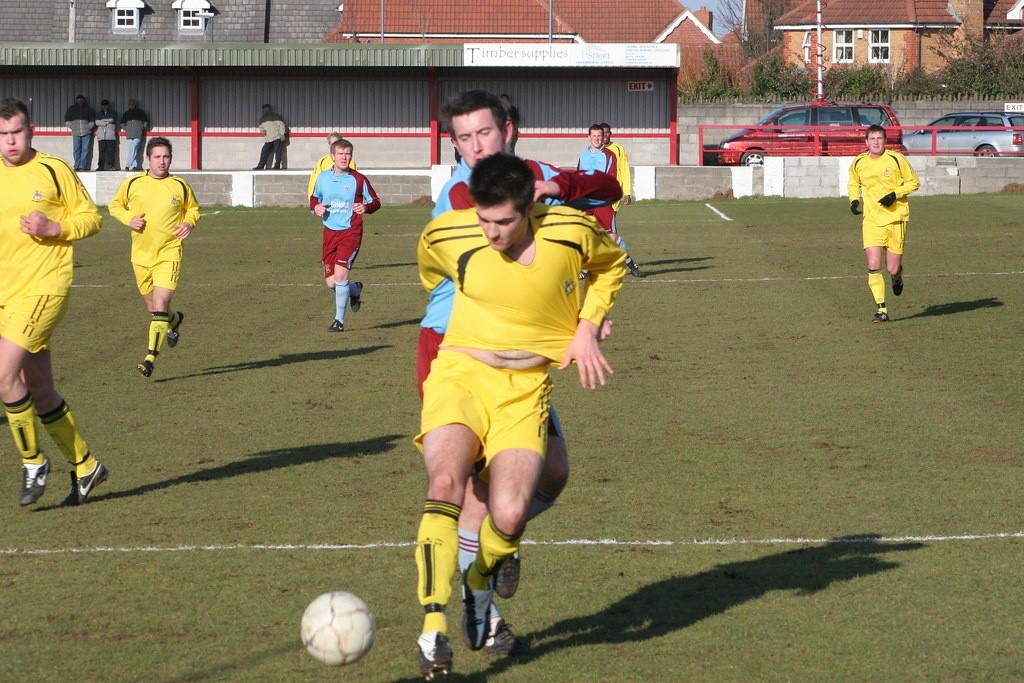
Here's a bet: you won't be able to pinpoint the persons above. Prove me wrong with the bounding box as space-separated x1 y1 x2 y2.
94 99 117 171
119 99 150 171
253 104 286 171
847 123 921 322
0 95 103 508
411 87 627 683
64 95 94 172
108 137 201 378
576 124 640 277
308 132 357 204
599 121 631 235
310 139 381 332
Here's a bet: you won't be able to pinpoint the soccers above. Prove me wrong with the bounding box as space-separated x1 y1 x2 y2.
300 590 376 666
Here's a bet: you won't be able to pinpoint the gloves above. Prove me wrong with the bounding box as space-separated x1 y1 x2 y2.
879 192 896 207
850 200 862 216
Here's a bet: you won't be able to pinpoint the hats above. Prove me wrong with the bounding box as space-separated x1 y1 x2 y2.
76 95 85 100
101 100 109 105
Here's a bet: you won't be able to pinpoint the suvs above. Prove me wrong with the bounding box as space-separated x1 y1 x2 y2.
901 111 1024 157
717 100 903 167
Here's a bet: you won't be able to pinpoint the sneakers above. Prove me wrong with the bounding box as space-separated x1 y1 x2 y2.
872 311 890 323
327 319 344 332
137 360 153 378
494 553 520 599
167 311 184 348
460 562 494 652
60 461 109 508
579 270 588 279
19 458 50 505
350 281 363 313
416 631 453 680
629 260 640 277
483 619 530 656
891 265 903 296
